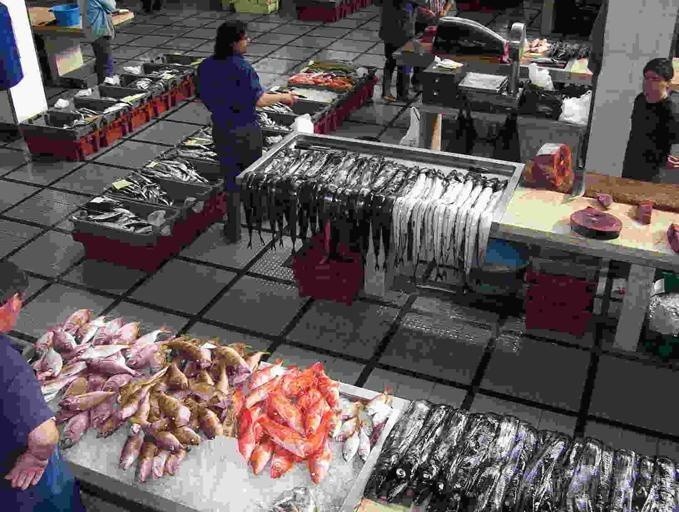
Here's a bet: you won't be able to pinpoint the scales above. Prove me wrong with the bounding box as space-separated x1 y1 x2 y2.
459 22 526 110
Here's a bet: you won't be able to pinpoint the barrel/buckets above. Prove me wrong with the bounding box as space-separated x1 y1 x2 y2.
49 4 80 26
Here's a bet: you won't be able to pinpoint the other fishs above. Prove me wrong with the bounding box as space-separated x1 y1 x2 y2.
111 329 272 481
362 384 679 512
31 307 170 456
224 351 392 482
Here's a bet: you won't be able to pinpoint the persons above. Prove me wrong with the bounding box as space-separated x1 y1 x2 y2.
140 0 164 15
377 0 418 102
74 1 121 83
410 0 458 96
0 259 86 511
620 56 679 183
195 21 301 240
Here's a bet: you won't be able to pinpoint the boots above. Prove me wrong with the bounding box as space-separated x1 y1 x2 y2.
224 193 242 243
397 73 413 103
383 75 398 102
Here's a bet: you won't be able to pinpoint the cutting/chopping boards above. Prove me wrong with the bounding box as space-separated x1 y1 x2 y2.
571 209 623 240
